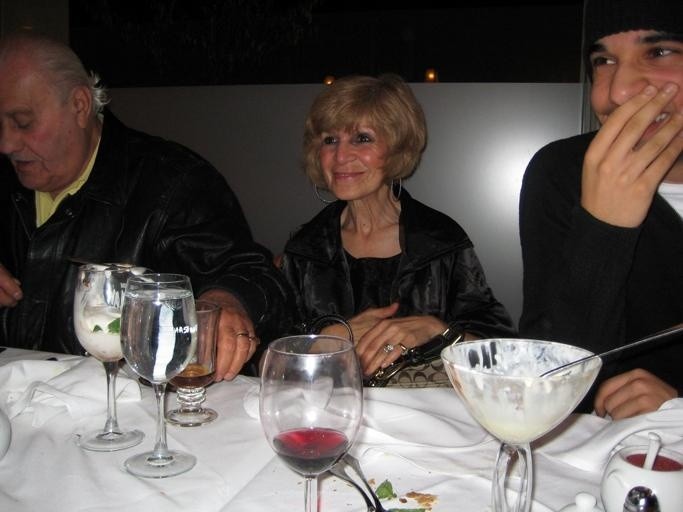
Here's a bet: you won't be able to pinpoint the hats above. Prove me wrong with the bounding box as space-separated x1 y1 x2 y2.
573 0 682 68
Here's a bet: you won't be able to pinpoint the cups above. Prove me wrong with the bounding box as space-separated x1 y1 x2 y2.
599 443 683 512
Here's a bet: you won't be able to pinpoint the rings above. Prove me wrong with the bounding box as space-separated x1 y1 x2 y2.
384 343 393 352
397 342 407 351
234 329 249 338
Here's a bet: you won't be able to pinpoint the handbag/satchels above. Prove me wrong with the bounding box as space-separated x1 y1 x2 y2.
368 323 464 390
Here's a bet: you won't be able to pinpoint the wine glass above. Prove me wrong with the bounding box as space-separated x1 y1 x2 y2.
72 261 145 454
118 273 199 480
439 337 604 512
258 332 366 512
165 299 223 429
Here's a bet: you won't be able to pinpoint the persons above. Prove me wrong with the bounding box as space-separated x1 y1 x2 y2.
0 29 280 389
514 0 683 423
233 71 518 393
